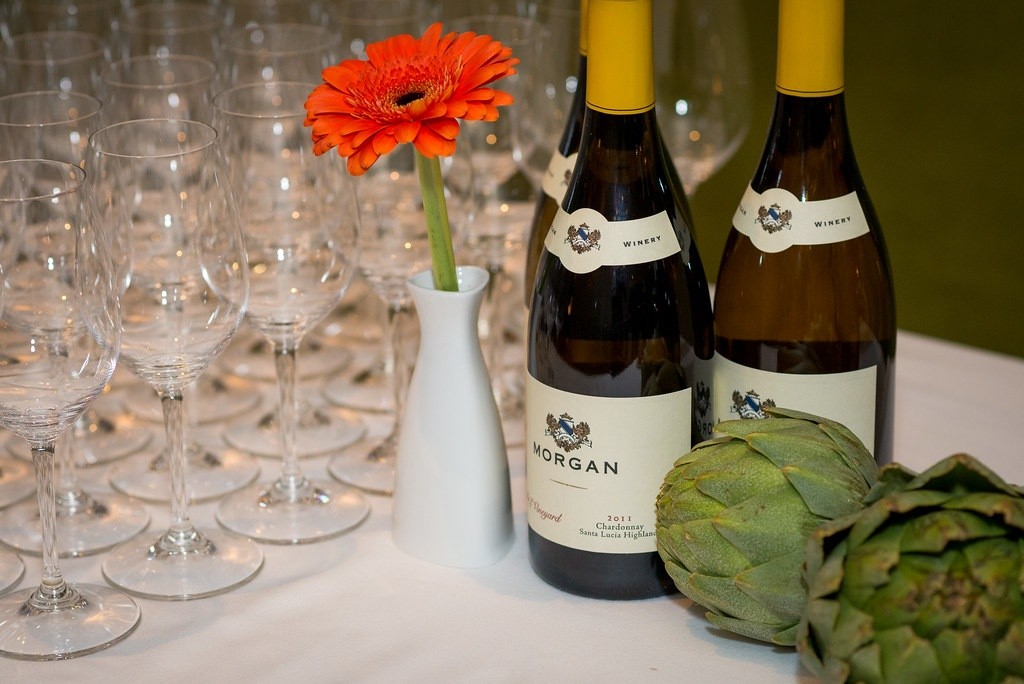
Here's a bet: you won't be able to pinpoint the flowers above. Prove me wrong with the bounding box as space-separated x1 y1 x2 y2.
305 21 520 291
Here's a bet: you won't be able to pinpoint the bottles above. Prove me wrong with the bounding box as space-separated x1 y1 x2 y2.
526 0 714 600
715 0 898 462
526 0 587 321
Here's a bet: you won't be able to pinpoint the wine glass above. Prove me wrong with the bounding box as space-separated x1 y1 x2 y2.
0 0 757 659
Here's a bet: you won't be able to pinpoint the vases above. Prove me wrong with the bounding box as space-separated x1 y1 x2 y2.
391 266 518 570
